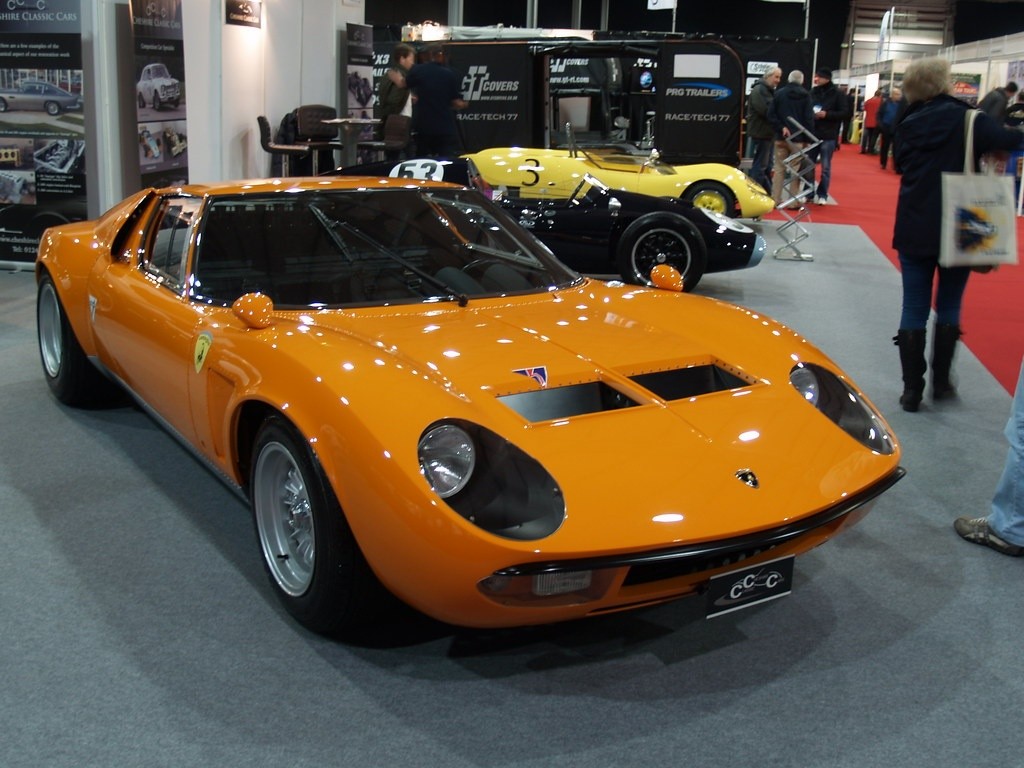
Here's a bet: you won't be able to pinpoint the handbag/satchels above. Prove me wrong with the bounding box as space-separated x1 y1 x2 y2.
939 173 1019 266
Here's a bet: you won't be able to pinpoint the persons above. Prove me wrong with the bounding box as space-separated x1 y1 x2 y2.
859 90 886 154
890 61 1024 409
773 70 812 209
975 82 1023 128
953 361 1024 559
377 41 467 162
875 88 902 170
750 65 784 194
803 68 846 204
841 87 857 144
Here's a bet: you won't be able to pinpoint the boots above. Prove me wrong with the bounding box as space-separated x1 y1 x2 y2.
893 329 927 412
932 321 964 401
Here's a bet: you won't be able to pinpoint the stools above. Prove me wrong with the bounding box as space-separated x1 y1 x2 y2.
359 113 415 173
258 115 311 178
292 105 347 175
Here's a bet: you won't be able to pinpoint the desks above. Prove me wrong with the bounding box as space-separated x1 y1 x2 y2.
320 117 382 174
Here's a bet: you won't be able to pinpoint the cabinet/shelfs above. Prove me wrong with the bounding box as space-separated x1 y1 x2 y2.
773 116 824 263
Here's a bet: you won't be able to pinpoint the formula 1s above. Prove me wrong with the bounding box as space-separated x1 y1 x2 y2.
138 129 163 160
157 126 188 157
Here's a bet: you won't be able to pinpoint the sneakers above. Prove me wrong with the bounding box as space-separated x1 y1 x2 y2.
955 515 1024 557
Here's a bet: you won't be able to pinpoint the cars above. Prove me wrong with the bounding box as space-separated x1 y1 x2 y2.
136 63 182 112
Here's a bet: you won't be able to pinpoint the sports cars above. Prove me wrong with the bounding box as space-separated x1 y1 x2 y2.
309 152 767 295
0 80 83 117
453 120 777 224
36 175 910 642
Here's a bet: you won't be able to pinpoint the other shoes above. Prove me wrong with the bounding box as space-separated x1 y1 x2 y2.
801 198 814 204
881 163 886 168
861 150 875 155
817 198 827 204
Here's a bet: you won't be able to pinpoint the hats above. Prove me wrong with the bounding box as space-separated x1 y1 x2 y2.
817 66 833 80
